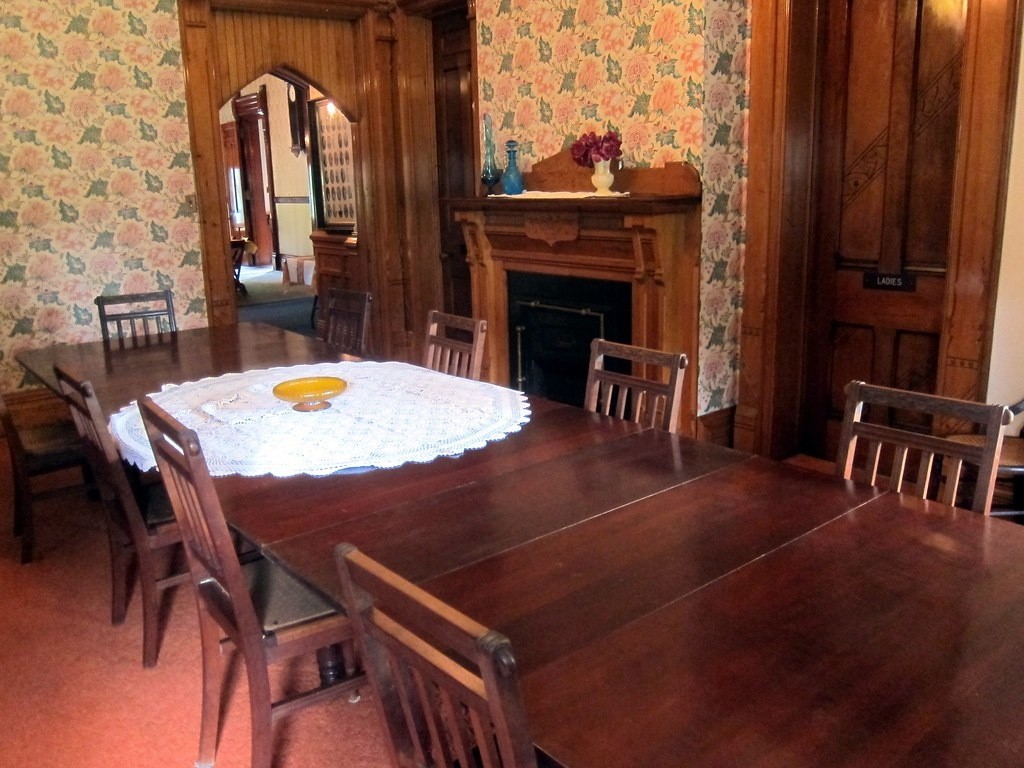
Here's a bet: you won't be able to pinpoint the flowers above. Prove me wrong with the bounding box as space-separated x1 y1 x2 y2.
570 130 623 168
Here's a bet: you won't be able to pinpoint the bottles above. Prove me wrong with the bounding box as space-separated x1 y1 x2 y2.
500 140 524 195
482 114 499 196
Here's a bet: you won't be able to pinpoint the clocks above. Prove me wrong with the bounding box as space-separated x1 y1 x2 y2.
286 82 306 158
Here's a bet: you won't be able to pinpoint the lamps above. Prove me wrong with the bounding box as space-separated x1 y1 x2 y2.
232 214 244 239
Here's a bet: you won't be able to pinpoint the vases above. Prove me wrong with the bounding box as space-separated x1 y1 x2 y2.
481 113 503 196
591 155 615 196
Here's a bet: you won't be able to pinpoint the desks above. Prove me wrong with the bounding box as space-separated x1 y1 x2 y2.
12 322 1024 768
280 255 319 331
231 239 258 297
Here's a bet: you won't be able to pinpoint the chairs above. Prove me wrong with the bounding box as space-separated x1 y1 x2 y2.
423 309 487 379
325 287 375 353
0 396 140 564
582 337 689 432
94 291 177 340
138 392 366 768
928 398 1024 526
832 380 1014 516
334 542 562 768
52 361 262 669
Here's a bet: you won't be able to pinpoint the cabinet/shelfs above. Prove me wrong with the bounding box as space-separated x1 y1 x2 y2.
309 228 362 350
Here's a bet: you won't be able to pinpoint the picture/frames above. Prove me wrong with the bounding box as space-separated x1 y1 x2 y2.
306 97 356 232
